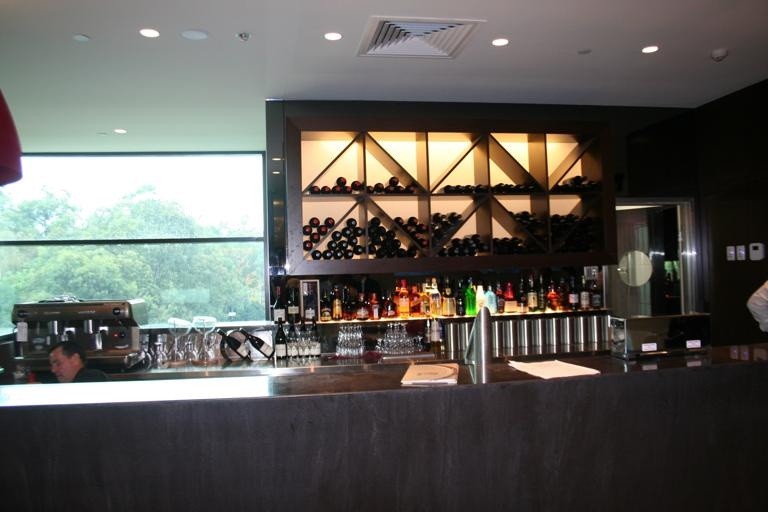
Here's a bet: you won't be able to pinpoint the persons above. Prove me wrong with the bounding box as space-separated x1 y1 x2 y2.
747 281 768 333
47 340 109 382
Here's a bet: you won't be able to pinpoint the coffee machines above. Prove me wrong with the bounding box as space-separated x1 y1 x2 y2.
11 299 149 374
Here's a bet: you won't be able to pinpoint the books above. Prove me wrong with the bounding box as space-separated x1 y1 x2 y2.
401 364 460 385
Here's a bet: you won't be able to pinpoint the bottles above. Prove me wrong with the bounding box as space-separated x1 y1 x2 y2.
422 315 440 344
275 320 321 362
304 283 316 322
307 174 600 196
287 287 300 324
320 267 601 324
238 328 275 358
301 210 600 260
272 286 286 324
217 329 250 360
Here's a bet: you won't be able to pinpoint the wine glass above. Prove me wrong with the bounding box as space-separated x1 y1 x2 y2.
147 316 218 372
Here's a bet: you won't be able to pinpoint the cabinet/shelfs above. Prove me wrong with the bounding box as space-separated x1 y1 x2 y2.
284 116 618 276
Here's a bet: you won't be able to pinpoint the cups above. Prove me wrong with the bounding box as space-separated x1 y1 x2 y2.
445 314 602 355
372 320 422 357
335 325 365 358
